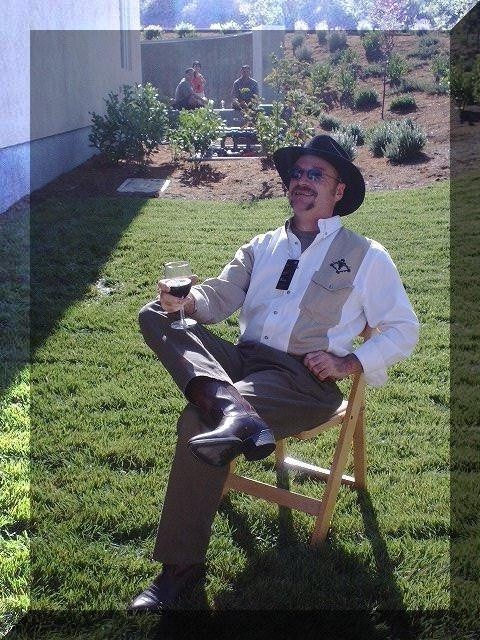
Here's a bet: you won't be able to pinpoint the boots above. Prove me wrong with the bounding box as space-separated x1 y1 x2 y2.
187 381 276 468
127 565 203 611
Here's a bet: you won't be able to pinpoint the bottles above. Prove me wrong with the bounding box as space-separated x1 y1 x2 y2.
220 100 226 110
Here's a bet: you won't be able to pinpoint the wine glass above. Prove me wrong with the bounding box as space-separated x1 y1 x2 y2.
164 261 198 330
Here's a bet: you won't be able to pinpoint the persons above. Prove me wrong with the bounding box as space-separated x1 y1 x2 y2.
174 68 209 110
231 64 259 128
128 135 420 615
190 60 207 98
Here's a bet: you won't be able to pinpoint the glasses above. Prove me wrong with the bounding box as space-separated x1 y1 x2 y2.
289 166 343 183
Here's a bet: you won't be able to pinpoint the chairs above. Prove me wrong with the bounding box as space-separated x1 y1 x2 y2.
220 322 380 549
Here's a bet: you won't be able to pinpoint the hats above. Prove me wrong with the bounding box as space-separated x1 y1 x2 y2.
273 135 365 216
